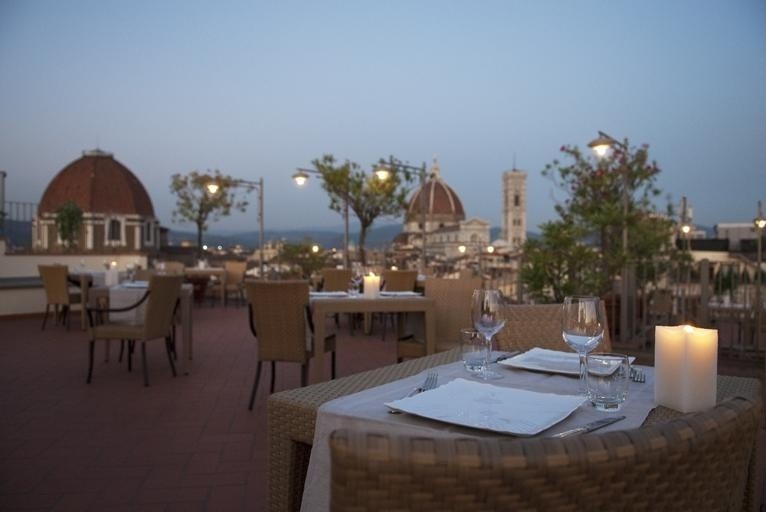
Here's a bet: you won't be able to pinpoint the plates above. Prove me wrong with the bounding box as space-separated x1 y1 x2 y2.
496 345 638 377
380 291 422 295
309 290 348 297
384 377 592 438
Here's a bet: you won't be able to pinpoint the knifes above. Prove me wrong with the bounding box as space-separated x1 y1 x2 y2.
552 413 625 439
487 350 522 364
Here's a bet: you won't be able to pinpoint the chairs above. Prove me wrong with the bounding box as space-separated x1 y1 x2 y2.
324 395 763 512
488 298 612 357
35 258 494 413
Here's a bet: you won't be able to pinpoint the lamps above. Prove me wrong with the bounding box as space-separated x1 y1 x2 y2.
655 322 718 417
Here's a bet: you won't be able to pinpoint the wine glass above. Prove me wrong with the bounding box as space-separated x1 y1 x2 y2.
563 295 607 409
469 289 506 381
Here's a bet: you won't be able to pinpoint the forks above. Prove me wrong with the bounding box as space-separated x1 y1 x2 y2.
628 365 647 383
388 371 439 414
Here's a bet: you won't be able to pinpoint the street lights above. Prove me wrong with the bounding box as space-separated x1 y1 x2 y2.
459 240 496 289
292 164 350 272
681 206 694 258
750 201 766 363
375 158 430 277
203 175 265 283
587 130 632 342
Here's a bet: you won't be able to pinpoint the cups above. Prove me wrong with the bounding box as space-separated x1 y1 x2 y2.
460 329 493 375
347 283 359 298
584 351 629 412
